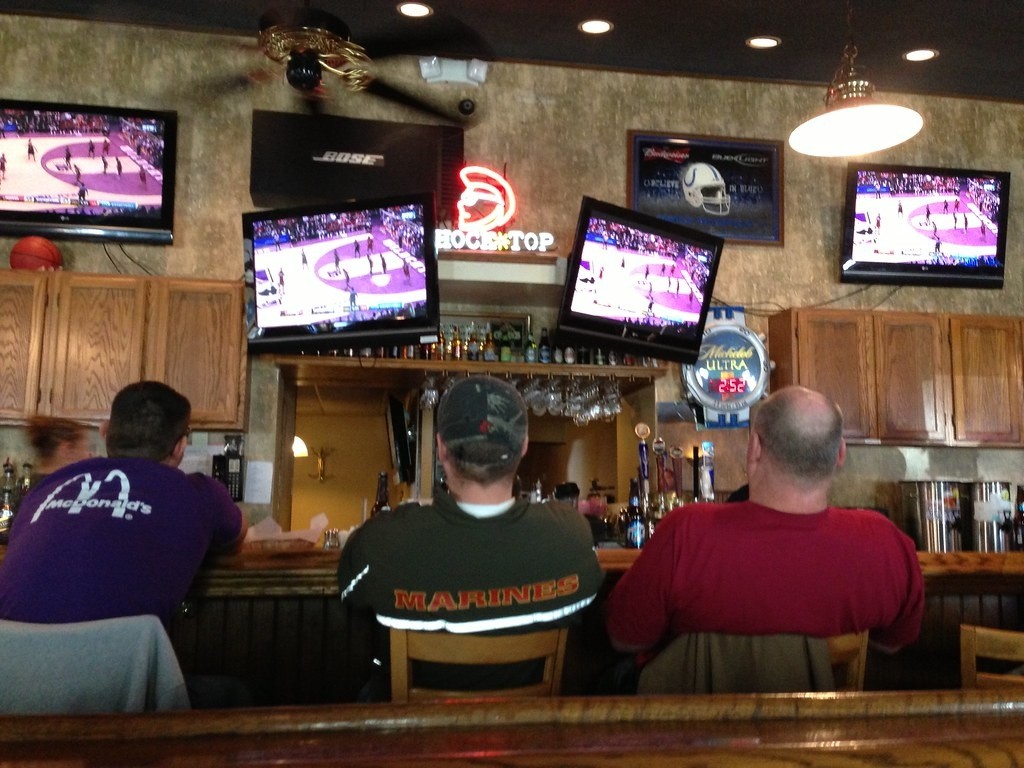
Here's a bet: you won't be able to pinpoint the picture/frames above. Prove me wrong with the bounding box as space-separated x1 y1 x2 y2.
627 129 785 248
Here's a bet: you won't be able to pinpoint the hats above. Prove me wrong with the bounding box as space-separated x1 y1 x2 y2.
438 375 527 465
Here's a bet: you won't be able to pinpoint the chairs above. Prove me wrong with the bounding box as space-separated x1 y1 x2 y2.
389 626 569 705
959 623 1024 690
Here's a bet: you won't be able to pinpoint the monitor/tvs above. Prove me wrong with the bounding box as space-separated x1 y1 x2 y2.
556 195 726 364
242 190 441 356
385 393 412 471
842 162 1010 290
0 98 178 245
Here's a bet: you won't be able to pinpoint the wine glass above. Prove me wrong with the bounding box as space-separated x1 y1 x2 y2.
419 370 624 426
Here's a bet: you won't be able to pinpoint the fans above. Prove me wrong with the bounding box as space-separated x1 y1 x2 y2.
191 1 493 132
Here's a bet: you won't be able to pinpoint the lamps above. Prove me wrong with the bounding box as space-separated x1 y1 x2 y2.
788 0 923 159
419 54 487 84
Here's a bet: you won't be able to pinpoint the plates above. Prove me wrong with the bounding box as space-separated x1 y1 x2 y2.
243 538 315 554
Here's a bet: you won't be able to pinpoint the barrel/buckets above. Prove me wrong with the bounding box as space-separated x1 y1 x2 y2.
962 481 1010 552
899 479 961 553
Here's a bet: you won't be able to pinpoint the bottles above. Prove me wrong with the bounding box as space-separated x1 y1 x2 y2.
1014 509 1024 550
0 463 35 541
323 528 340 550
626 477 646 551
292 313 659 369
368 471 392 518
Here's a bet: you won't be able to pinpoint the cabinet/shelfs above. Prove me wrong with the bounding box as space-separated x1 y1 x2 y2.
0 268 249 429
767 307 1024 448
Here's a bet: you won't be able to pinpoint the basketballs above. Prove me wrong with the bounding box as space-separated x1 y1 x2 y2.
11 236 65 271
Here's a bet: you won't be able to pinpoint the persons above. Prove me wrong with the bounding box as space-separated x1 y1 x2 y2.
606 385 927 689
0 381 247 626
858 171 1001 267
587 217 713 338
30 415 89 489
0 112 163 216
338 376 604 702
253 205 427 333
